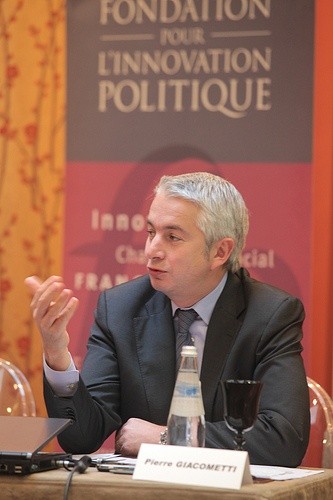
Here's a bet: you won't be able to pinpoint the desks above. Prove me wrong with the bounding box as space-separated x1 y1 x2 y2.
0 455 333 500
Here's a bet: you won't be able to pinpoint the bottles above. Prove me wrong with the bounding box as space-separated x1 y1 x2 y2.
167 346 205 448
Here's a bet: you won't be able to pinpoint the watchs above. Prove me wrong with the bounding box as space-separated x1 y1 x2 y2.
156 428 170 446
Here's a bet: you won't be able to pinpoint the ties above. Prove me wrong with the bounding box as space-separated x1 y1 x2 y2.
175 309 199 373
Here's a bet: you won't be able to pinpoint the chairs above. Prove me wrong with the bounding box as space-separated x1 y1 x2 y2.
0 358 36 416
300 376 333 470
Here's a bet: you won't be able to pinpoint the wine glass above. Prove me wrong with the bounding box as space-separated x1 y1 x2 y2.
219 379 263 451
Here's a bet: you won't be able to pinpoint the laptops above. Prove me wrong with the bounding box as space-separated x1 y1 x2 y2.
0 416 74 475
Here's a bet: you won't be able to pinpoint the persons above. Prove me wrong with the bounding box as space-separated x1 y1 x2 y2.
24 172 311 465
